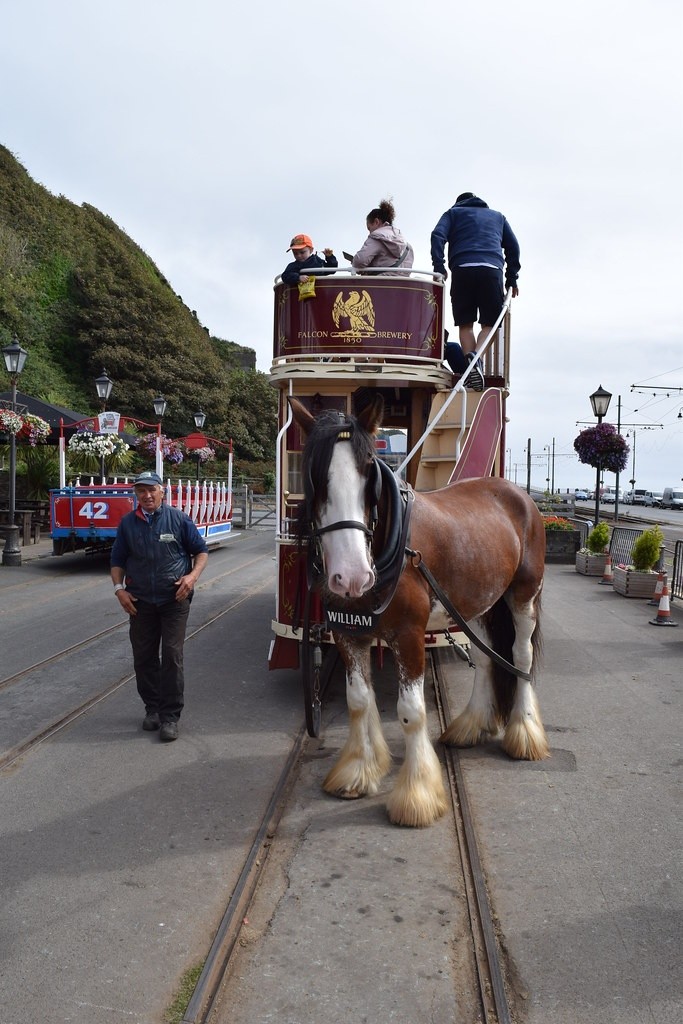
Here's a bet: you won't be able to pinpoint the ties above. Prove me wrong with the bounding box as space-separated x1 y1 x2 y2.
146 514 153 525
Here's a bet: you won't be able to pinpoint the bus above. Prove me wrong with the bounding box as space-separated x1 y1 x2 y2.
268 266 514 671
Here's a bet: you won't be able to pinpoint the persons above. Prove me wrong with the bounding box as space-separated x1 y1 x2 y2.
430 192 521 394
110 472 209 740
280 235 338 285
353 202 414 281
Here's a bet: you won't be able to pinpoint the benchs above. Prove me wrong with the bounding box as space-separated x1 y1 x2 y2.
0 498 51 546
0 517 50 546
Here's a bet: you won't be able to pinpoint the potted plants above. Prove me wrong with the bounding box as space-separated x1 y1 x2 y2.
614 523 664 598
576 521 610 576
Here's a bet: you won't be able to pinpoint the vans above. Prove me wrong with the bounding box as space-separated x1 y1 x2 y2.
644 491 664 508
662 487 683 510
594 485 648 506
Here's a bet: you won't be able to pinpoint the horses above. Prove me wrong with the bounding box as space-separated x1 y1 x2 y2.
285 387 550 826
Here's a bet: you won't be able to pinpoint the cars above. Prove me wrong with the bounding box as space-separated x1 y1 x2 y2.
587 491 595 500
601 493 615 505
575 491 588 501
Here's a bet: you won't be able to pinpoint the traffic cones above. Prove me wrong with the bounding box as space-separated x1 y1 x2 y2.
646 573 665 606
649 586 680 627
598 556 614 585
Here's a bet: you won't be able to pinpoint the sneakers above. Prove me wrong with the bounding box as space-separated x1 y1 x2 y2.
159 721 178 740
142 711 161 730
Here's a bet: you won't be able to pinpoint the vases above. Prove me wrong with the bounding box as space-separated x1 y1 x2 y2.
545 529 581 564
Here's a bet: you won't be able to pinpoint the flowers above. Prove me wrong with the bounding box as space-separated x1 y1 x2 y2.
574 423 630 473
0 409 24 435
68 420 130 458
542 516 575 529
186 445 215 462
19 414 53 446
135 433 183 466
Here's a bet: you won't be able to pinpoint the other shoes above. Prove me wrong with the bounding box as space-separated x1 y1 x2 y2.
466 351 484 393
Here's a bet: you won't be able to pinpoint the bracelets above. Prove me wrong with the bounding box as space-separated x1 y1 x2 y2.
114 584 124 595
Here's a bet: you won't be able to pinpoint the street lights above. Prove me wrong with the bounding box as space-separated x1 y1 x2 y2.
588 384 612 528
152 389 169 424
193 405 207 479
1 332 30 566
93 367 114 486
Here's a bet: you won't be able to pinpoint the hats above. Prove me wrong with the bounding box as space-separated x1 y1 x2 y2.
286 235 314 252
131 471 163 487
